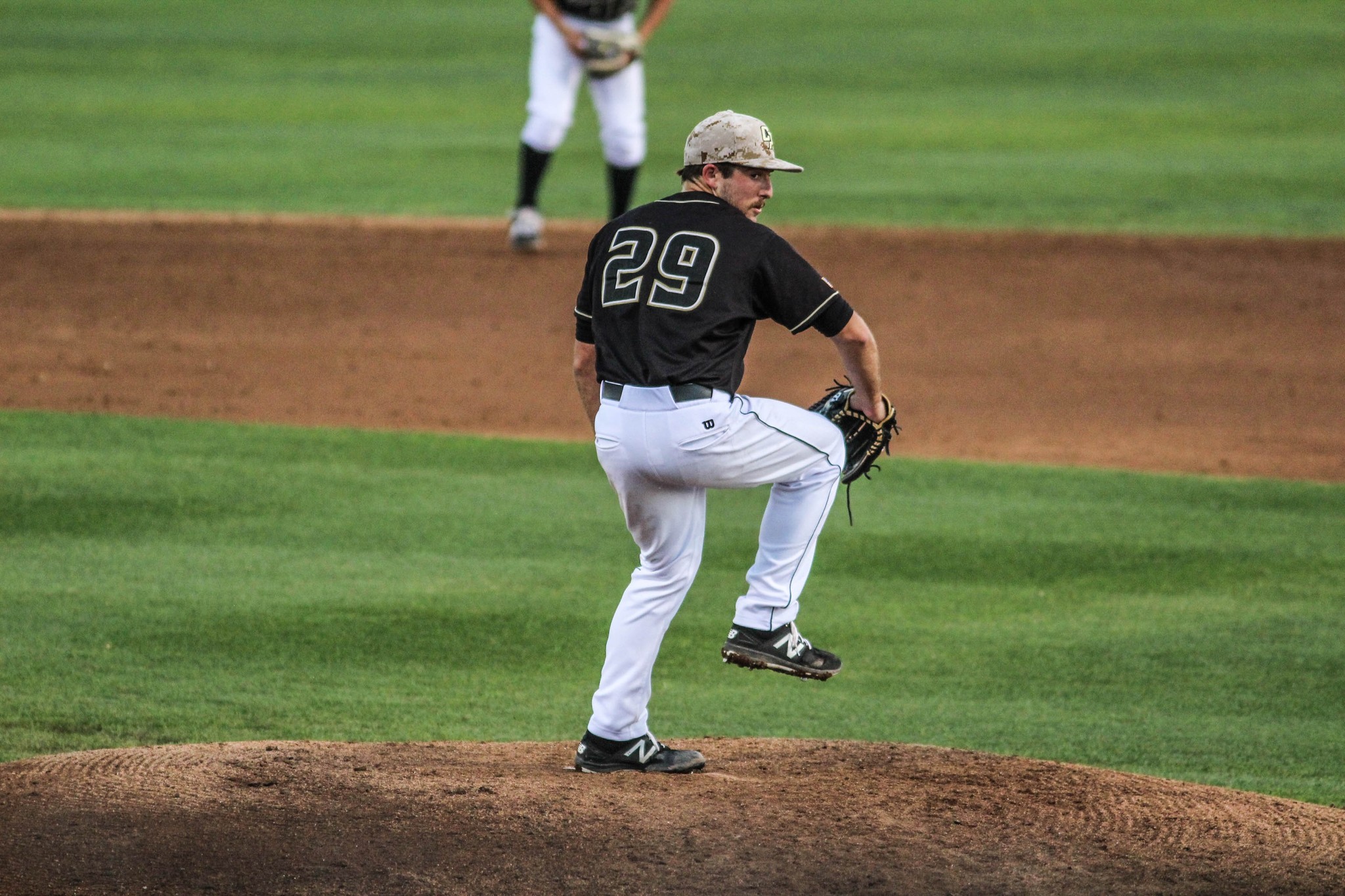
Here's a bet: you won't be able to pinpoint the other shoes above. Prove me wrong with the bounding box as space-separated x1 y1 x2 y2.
506 206 544 245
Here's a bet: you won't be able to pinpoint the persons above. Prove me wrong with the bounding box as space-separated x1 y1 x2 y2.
573 109 897 772
509 0 672 253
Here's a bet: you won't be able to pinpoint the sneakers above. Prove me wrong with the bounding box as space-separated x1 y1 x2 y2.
574 728 706 775
720 621 843 680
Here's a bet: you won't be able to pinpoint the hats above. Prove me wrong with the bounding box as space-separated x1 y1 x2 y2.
683 109 804 173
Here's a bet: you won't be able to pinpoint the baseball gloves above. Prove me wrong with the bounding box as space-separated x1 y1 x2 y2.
805 373 904 528
575 25 642 79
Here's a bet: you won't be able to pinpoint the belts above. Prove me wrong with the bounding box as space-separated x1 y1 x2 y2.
602 380 712 403
